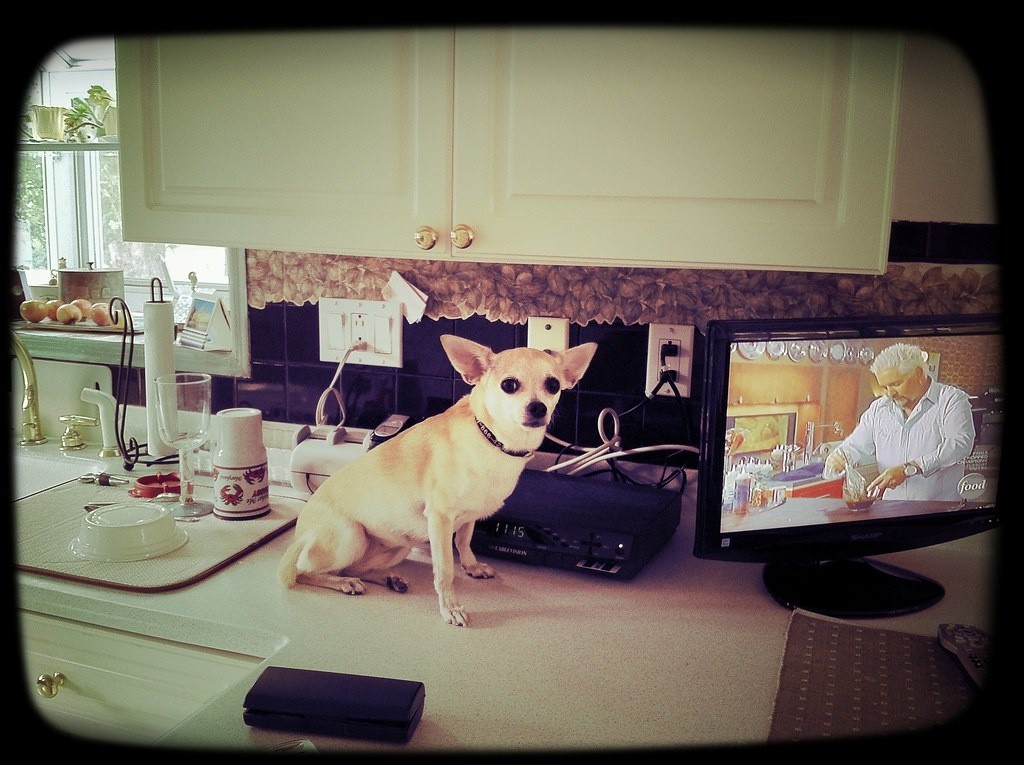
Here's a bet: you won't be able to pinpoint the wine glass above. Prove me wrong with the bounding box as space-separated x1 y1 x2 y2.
154 373 215 517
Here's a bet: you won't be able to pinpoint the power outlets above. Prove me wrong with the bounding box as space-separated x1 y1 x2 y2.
318 296 403 368
645 322 694 397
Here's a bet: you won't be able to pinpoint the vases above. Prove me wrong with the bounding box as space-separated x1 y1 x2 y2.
30 104 66 140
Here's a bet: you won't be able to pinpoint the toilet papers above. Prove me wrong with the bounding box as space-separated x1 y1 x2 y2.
143 300 179 460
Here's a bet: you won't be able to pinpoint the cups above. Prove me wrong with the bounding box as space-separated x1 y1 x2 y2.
212 407 272 521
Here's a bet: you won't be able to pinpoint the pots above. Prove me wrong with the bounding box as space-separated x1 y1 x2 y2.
57 262 125 312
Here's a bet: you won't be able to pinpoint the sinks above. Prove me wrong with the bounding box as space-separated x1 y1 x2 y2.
14 443 108 505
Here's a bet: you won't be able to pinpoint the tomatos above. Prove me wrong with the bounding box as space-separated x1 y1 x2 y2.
19 299 114 325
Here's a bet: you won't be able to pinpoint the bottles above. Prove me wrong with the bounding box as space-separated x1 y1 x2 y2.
721 444 785 516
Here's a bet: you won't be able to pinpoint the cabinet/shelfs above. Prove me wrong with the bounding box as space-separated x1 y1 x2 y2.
16 611 257 743
111 33 905 277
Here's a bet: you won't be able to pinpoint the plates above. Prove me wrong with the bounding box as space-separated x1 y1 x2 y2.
731 340 873 365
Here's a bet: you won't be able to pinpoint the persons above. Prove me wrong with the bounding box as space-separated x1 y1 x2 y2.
826 341 976 501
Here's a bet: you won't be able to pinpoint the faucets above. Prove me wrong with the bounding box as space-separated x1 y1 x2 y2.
8 328 48 446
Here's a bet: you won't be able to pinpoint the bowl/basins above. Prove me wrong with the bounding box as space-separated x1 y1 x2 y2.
128 473 196 500
842 485 881 511
69 502 188 562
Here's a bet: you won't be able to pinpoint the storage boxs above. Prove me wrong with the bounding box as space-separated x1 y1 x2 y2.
243 665 425 745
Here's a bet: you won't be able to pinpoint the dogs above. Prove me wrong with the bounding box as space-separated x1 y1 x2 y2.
279 334 598 626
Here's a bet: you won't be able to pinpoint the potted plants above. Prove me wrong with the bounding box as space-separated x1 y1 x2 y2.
63 85 116 143
